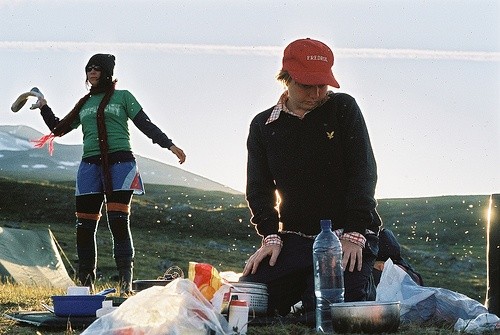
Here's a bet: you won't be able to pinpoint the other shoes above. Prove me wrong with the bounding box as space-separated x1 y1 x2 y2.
374 260 421 287
298 272 317 312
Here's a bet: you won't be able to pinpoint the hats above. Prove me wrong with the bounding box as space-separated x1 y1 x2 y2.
282 37 339 88
85 53 115 75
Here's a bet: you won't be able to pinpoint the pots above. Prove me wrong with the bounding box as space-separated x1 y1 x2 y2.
51 289 115 317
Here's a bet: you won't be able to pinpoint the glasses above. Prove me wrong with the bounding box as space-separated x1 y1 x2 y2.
87 64 102 72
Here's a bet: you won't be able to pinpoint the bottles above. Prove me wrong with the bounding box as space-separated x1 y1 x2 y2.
313 218 344 333
227 300 249 333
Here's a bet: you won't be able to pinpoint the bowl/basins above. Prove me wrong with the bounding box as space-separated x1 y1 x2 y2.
329 301 401 332
223 281 270 314
132 280 170 291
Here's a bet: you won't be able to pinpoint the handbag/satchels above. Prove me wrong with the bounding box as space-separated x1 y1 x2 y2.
78 278 236 335
374 259 490 323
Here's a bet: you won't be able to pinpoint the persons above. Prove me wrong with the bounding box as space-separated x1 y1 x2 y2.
36 54 186 298
239 38 424 327
